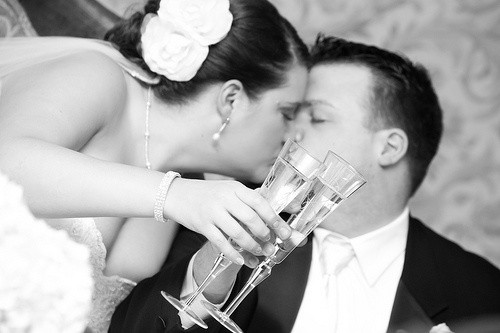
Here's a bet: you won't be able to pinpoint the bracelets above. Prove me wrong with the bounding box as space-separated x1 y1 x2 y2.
154 171 181 222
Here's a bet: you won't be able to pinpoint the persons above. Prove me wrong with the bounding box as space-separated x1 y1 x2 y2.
107 32 500 333
0 0 310 332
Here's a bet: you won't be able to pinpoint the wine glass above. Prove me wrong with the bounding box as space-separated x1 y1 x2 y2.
201 150 367 333
161 139 327 329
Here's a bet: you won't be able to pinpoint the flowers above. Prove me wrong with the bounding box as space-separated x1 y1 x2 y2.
140 0 233 81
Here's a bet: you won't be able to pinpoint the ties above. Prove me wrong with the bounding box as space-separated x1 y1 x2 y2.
291 240 357 333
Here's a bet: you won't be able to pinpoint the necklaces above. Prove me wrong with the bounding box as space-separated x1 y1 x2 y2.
144 86 153 168
